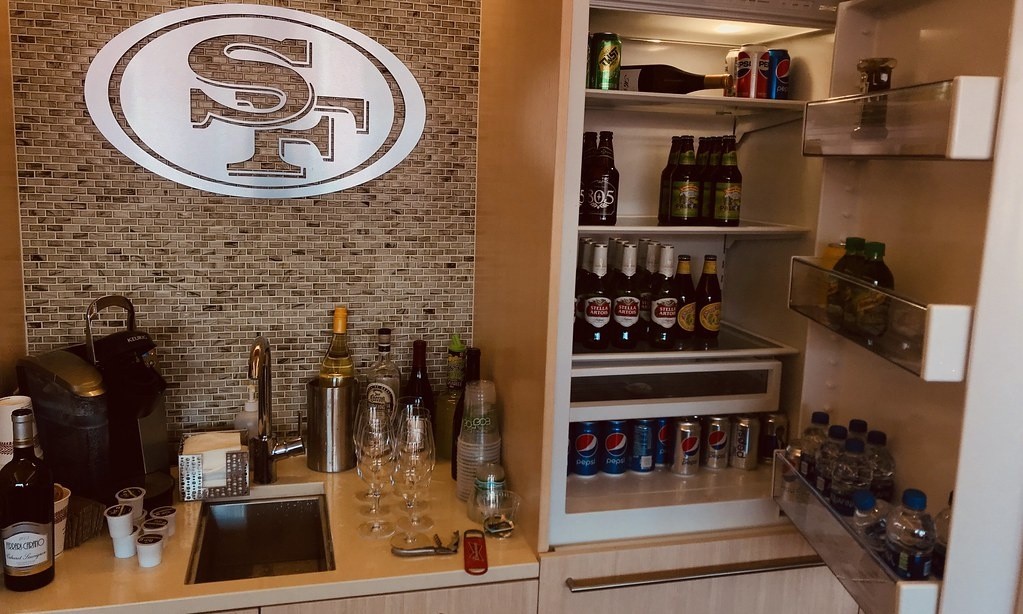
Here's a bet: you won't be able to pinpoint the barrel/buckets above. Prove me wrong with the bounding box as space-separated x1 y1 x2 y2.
305 376 360 474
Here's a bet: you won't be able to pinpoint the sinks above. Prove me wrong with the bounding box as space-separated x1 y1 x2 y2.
181 479 339 587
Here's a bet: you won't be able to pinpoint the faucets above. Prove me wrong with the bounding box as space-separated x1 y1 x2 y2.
246 337 305 485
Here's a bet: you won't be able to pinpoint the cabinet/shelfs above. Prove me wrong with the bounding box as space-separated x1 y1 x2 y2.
535 523 861 614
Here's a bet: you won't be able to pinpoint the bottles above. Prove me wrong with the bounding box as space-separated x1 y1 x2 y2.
435 333 467 462
318 307 354 388
467 461 510 524
578 131 620 226
800 411 954 581
572 236 723 354
657 134 742 228
451 348 481 481
620 64 734 95
401 340 434 454
0 408 56 592
366 327 401 456
825 236 894 338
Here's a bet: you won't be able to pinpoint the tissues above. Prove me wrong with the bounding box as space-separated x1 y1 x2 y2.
178 431 252 503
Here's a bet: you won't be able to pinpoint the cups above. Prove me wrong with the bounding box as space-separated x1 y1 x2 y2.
0 395 44 469
455 379 501 502
53 488 71 560
104 486 177 567
477 488 522 541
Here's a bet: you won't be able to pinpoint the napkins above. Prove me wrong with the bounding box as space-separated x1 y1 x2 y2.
181 431 248 487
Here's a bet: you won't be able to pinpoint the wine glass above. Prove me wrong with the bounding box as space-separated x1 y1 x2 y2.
352 396 434 550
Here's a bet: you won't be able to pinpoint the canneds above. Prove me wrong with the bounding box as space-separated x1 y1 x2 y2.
671 412 788 477
570 418 674 477
587 31 622 90
722 43 790 100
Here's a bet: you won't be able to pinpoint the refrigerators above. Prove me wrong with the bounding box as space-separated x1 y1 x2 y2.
548 0 1023 613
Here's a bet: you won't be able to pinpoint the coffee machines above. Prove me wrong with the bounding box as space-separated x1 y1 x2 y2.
14 287 180 518
